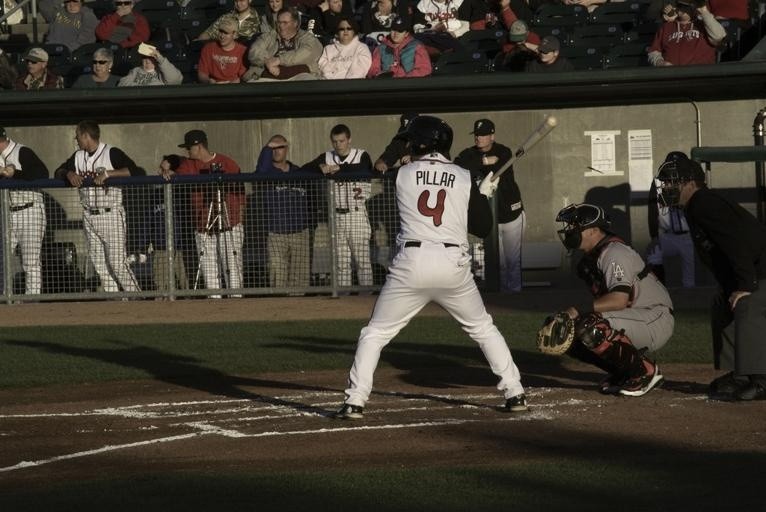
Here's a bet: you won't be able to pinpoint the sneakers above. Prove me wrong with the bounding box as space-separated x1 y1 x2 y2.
737 381 766 399
619 360 663 397
508 394 527 412
600 372 624 394
334 402 363 420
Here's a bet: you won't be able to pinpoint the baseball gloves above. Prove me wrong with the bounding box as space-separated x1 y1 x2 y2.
536 312 575 356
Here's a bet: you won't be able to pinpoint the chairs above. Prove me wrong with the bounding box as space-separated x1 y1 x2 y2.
433 3 656 77
18 12 217 88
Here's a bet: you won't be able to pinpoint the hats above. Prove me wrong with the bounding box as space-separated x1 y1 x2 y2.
391 16 408 32
398 113 418 131
539 35 560 53
24 47 48 62
178 130 206 147
469 119 495 135
219 18 237 33
509 20 528 42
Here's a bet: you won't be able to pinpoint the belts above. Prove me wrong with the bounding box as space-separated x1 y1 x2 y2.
9 203 33 211
90 208 110 214
405 242 457 247
335 208 358 213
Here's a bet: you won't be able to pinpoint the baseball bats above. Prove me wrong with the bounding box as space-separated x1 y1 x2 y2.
490 115 559 183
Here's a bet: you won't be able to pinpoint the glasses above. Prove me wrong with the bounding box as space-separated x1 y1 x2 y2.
92 60 107 64
339 27 353 30
115 1 131 6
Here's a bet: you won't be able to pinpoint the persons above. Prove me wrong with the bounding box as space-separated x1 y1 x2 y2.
0 127 49 295
655 154 766 403
461 118 527 292
642 151 698 291
55 124 143 300
1 1 765 92
535 202 677 398
330 111 531 421
255 113 422 288
151 128 245 299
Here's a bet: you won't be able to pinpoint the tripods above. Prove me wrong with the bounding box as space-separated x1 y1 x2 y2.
193 162 245 297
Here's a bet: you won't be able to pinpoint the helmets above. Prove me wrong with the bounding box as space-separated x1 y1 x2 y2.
655 157 705 207
396 116 453 157
556 203 608 250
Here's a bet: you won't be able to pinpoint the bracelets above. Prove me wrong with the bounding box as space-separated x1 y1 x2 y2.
573 299 594 317
